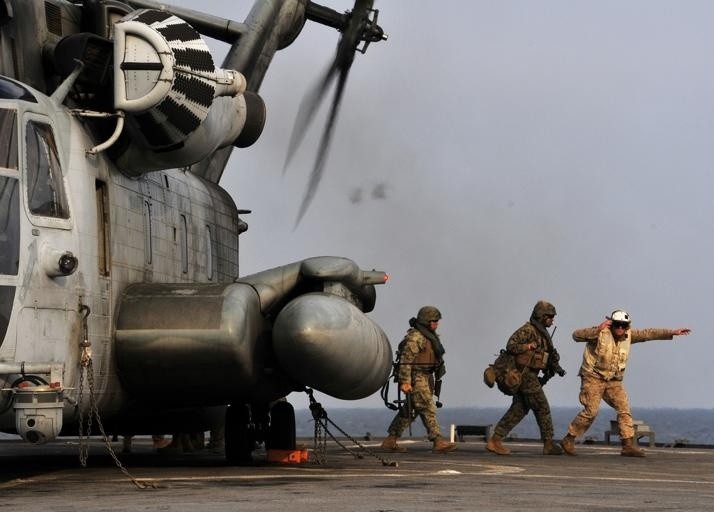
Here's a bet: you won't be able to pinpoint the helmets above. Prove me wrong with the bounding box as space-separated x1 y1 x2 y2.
611 310 630 323
416 306 441 325
533 301 556 318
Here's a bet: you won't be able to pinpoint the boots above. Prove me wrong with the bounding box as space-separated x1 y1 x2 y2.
431 437 456 453
486 433 511 454
542 439 565 455
621 437 646 457
559 432 577 455
381 433 406 453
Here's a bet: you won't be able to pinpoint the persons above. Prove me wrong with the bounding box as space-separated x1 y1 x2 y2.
485 301 567 456
559 310 692 458
379 306 459 455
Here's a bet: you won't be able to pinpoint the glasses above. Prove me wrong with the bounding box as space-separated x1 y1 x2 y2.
613 320 629 329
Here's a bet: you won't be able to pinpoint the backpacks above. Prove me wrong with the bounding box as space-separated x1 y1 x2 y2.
492 349 535 395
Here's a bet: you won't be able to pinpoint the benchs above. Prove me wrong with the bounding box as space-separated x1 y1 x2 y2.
604 430 656 446
449 425 496 441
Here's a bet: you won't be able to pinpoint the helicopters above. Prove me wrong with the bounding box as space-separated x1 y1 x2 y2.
1 1 392 463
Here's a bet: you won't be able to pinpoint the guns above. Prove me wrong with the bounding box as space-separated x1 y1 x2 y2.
537 327 566 387
394 391 414 437
